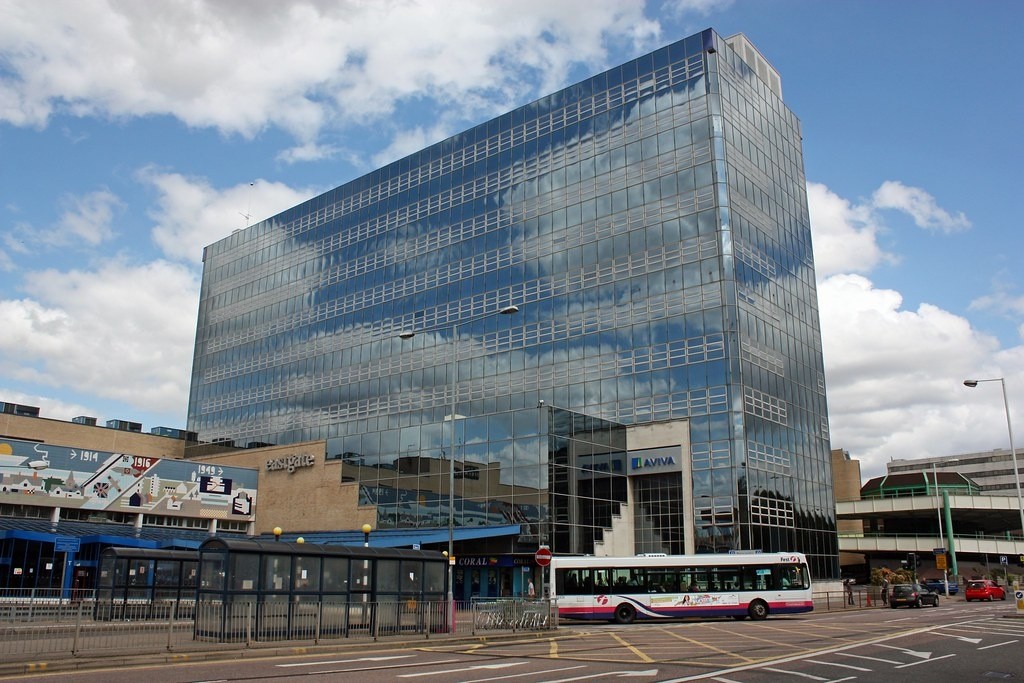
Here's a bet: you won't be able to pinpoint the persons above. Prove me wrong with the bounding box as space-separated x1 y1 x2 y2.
748 574 776 590
846 578 854 605
722 581 740 591
648 576 700 592
527 578 535 602
881 574 888 606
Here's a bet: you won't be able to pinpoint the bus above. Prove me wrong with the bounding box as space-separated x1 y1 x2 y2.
549 550 815 624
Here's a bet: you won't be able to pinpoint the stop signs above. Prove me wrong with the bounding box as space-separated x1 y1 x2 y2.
536 548 552 567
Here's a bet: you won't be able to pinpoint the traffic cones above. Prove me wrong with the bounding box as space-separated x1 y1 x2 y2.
865 595 874 608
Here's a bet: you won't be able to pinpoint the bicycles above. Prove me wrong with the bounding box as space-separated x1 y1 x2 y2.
475 603 552 632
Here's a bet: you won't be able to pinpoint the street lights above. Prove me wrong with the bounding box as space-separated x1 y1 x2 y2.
909 552 917 583
396 304 520 634
964 378 1024 541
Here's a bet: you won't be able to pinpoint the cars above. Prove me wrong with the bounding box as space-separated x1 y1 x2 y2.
889 583 940 609
925 577 959 595
965 579 1007 602
